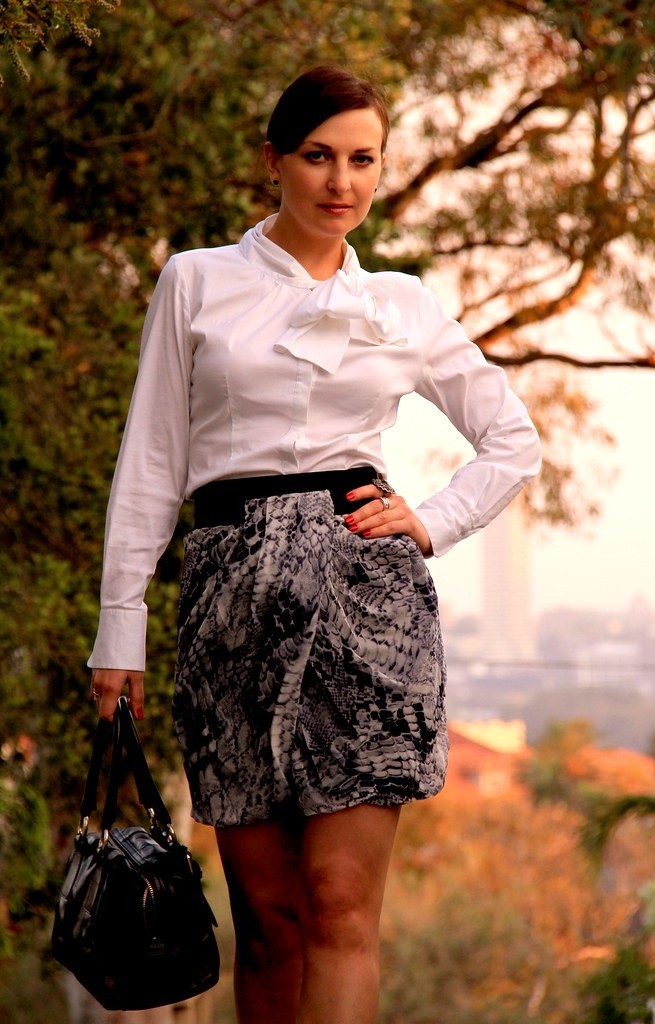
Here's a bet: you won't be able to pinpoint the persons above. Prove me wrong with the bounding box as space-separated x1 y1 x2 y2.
84 64 546 1023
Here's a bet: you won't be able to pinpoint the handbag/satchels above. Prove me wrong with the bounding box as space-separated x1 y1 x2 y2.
51 695 218 1011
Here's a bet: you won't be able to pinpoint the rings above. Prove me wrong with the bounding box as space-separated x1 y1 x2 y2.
379 495 390 511
372 477 395 497
91 688 101 702
